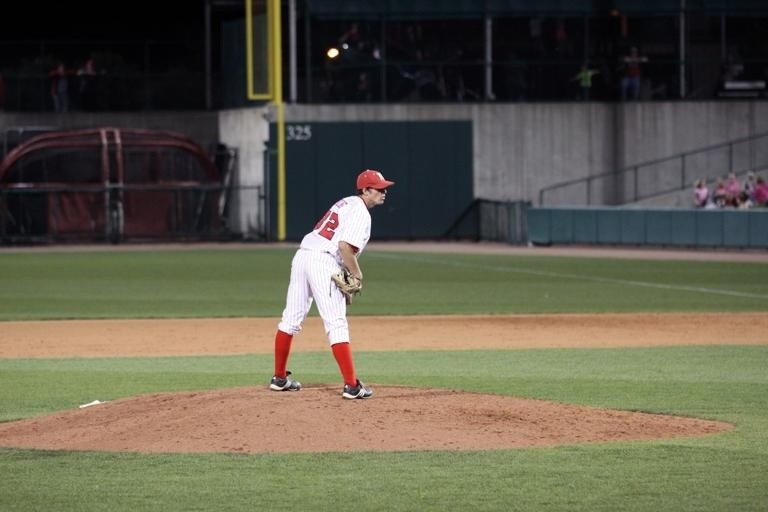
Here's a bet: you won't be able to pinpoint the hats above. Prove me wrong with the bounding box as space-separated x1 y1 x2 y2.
357 171 393 189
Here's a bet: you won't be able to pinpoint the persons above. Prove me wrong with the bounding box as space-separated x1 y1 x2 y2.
693 168 768 210
269 169 394 399
3 3 766 117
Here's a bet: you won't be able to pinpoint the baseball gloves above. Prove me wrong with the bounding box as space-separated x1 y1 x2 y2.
331 267 361 305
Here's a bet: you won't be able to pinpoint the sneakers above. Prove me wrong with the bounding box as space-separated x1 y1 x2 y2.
342 380 373 399
270 372 301 391
